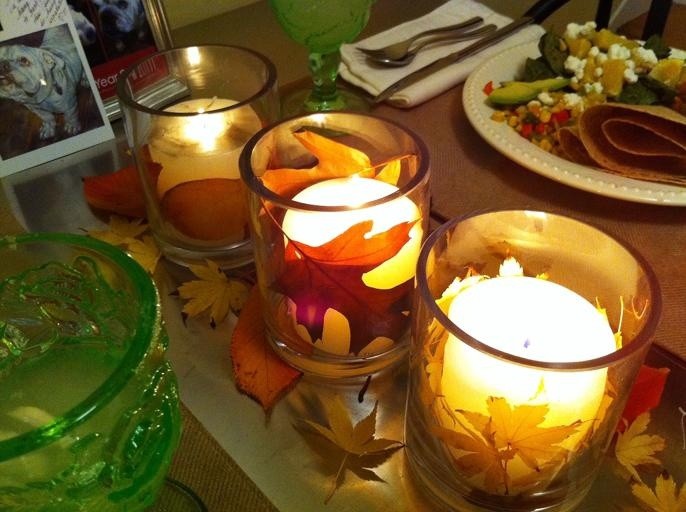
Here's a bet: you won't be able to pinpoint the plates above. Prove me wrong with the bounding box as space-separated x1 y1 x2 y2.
463 39 686 210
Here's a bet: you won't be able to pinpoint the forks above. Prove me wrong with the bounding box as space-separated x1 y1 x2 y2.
355 18 495 60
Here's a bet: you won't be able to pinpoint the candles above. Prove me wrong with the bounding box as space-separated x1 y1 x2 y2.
281 174 425 374
146 96 262 250
438 275 619 491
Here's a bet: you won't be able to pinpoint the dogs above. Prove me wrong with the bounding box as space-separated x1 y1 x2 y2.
93 0 148 53
0 23 90 140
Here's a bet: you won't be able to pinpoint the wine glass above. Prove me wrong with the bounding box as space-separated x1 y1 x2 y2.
270 0 372 134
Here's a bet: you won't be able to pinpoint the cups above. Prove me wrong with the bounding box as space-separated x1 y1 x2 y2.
118 42 279 267
402 209 663 510
237 115 428 382
1 230 189 511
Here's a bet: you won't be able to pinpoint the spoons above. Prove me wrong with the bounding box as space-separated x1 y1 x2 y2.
367 26 496 66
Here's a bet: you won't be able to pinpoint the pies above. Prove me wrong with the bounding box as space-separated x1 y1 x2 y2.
556 102 686 187
